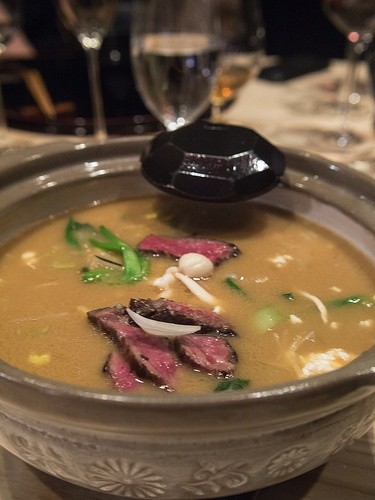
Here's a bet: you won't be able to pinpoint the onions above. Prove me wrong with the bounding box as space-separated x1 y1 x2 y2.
127 308 201 337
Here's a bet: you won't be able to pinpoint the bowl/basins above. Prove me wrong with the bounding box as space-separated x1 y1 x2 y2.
0 135 375 500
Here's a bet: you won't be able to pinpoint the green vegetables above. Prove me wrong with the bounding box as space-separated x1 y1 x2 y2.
326 294 372 310
64 217 148 283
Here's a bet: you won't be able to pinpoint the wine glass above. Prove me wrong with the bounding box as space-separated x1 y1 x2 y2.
58 0 122 142
316 0 375 154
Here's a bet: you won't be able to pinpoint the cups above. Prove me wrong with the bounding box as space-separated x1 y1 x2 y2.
209 0 262 122
131 0 226 133
